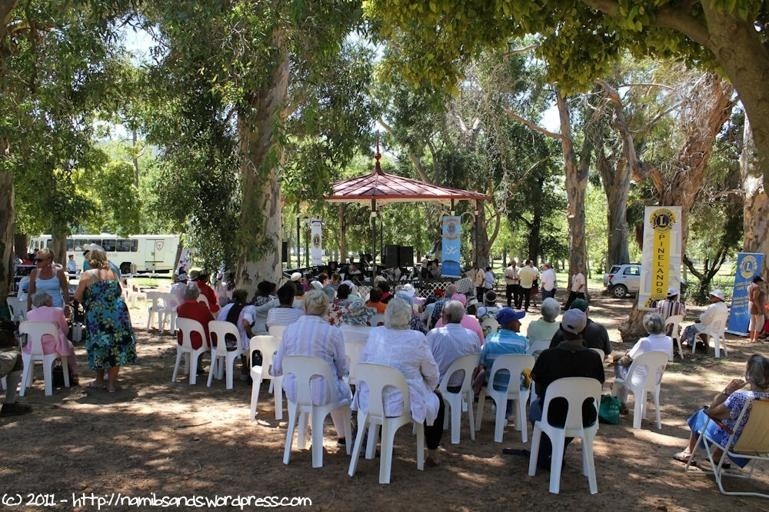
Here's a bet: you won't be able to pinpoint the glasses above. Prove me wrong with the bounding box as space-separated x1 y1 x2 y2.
35 258 44 262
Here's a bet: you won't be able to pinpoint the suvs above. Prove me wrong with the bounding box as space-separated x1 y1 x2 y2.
603 263 690 300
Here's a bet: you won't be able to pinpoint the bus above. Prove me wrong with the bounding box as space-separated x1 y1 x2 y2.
32 233 182 273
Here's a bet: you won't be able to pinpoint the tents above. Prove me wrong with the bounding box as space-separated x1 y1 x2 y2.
322 129 492 288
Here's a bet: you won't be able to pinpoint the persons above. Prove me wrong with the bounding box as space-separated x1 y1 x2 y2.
504 260 521 309
400 283 421 305
341 298 377 328
656 287 685 338
270 289 356 448
176 282 218 376
73 248 138 393
481 266 495 292
393 290 428 336
477 290 502 338
674 289 729 350
83 243 121 281
746 274 767 344
425 299 482 395
526 296 561 360
561 265 586 312
479 306 530 432
422 294 438 313
349 294 446 469
22 292 81 386
168 274 189 305
327 283 352 326
190 270 220 315
672 353 769 470
548 296 612 361
178 265 186 275
614 311 670 415
15 246 37 276
473 266 486 304
463 262 486 282
429 283 457 330
265 284 306 335
528 260 539 309
419 254 440 281
253 279 276 306
66 254 77 280
0 262 35 419
529 308 605 475
363 286 389 315
514 260 533 313
305 262 365 287
375 280 394 305
26 247 71 319
433 292 485 346
539 262 558 301
212 288 256 385
285 272 306 295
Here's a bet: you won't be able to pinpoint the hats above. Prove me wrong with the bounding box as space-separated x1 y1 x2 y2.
343 301 376 324
310 280 323 288
495 308 526 323
667 288 682 298
563 309 588 333
292 272 302 281
190 270 212 277
710 290 724 300
177 273 188 280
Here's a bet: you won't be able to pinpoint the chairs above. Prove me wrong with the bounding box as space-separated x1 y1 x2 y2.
8 297 24 320
664 315 684 359
267 325 288 394
475 353 535 444
204 320 249 391
692 315 729 358
172 315 210 386
144 291 163 334
527 375 603 496
158 293 177 334
16 321 71 398
346 364 426 485
688 390 769 498
611 351 668 432
247 335 285 421
281 358 352 467
526 339 552 406
588 347 605 363
439 353 481 445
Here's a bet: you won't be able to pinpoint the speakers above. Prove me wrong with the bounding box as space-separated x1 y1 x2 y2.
384 244 400 266
399 246 413 267
